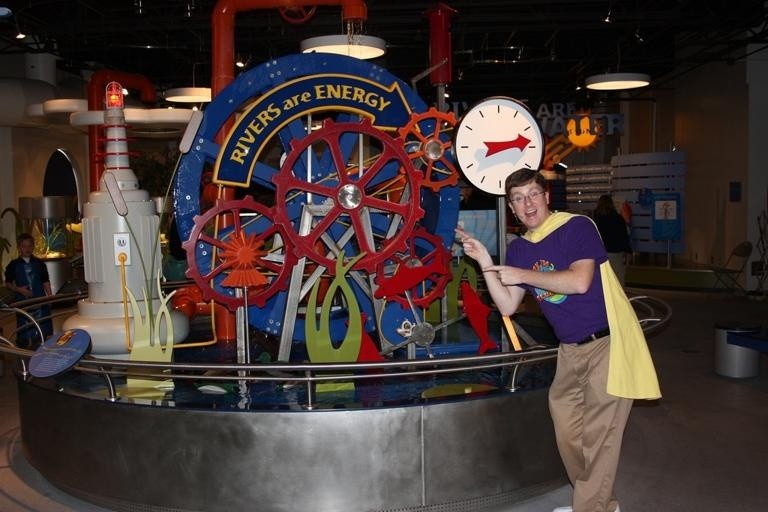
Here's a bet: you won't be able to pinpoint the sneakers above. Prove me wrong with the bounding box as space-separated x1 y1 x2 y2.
552 501 621 512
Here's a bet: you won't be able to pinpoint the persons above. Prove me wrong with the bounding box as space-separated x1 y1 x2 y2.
3 233 54 346
454 166 642 512
592 194 633 293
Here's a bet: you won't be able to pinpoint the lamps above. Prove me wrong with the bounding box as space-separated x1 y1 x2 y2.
299 1 388 61
583 28 653 93
162 63 214 104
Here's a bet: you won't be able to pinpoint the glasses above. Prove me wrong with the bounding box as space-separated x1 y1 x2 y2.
510 191 546 202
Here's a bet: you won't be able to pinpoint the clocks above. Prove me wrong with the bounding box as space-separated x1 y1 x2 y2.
450 94 547 198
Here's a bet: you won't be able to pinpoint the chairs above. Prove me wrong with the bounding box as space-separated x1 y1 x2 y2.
707 241 754 299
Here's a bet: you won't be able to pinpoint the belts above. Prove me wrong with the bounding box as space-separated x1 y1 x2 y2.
577 326 610 348
